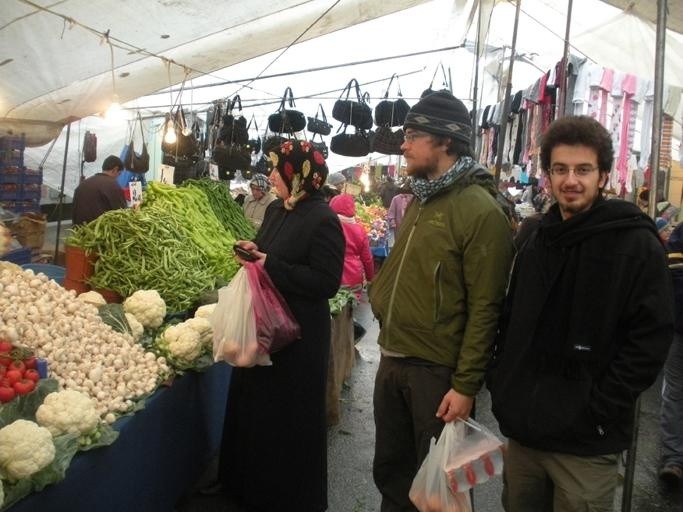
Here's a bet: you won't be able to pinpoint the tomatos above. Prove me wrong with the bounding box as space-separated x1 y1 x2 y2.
0 167 34 174
0 183 38 192
0 338 40 403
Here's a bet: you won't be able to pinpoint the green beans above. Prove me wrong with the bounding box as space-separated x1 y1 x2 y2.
56 207 219 318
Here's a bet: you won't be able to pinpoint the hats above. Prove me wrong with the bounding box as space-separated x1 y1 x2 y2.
404 91 474 145
654 215 666 233
327 172 347 185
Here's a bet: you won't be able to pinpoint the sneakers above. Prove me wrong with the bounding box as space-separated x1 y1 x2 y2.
197 484 222 498
658 467 681 485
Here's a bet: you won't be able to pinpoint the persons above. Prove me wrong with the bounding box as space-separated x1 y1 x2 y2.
242 174 376 344
71 157 128 222
363 89 518 512
485 116 676 511
659 221 683 478
380 176 551 241
640 190 676 243
218 141 346 512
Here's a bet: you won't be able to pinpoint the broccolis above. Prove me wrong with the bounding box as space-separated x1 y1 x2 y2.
0 418 55 481
164 304 219 367
35 391 99 447
77 289 168 340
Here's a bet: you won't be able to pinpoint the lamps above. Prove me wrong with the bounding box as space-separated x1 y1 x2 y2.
165 62 177 144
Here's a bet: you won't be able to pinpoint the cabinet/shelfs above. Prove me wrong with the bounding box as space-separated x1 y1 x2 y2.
101 34 130 142
327 300 355 425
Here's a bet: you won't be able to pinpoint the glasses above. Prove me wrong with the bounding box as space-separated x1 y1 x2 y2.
547 164 600 176
402 134 429 143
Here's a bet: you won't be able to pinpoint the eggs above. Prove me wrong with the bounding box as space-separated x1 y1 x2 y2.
235 353 252 367
245 341 258 359
223 341 242 362
410 489 462 512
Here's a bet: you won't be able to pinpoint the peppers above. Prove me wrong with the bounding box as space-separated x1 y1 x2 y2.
139 181 238 281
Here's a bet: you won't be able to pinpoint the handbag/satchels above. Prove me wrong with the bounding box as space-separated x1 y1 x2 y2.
262 137 291 161
124 112 150 173
268 111 306 134
204 95 261 182
308 132 328 161
161 105 209 185
419 64 452 99
330 73 410 156
114 122 149 191
308 103 333 135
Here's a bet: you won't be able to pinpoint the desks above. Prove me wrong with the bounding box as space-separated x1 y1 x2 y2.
370 244 387 270
0 362 232 512
